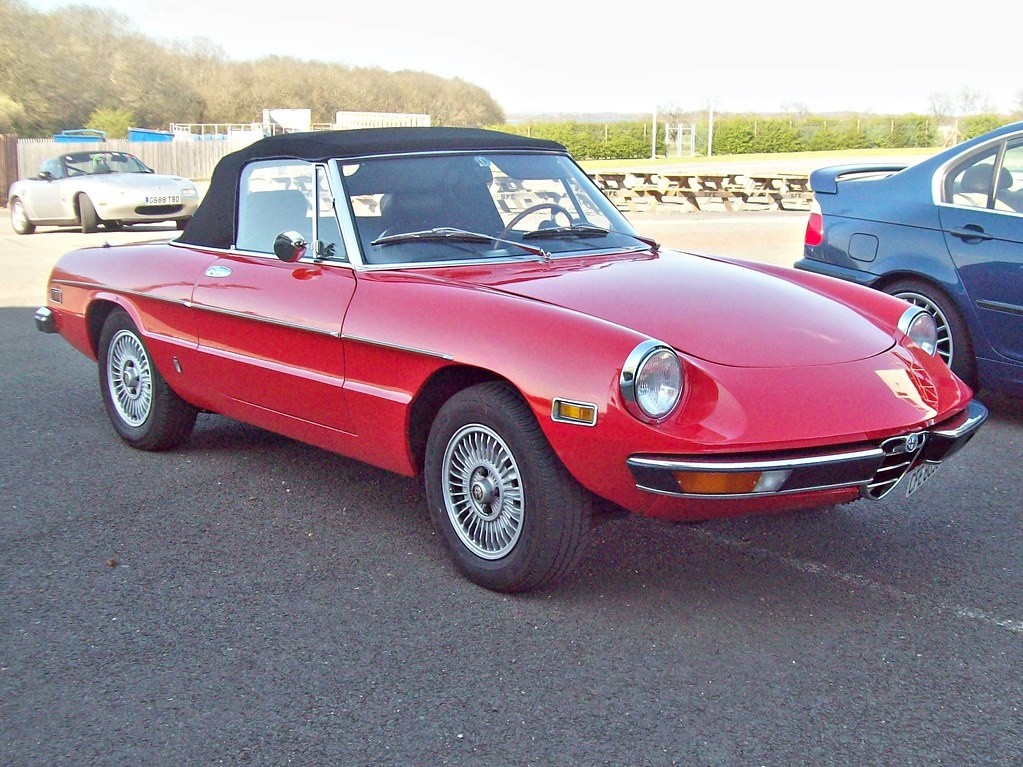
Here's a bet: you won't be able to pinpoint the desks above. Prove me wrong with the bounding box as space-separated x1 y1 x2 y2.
488 173 812 216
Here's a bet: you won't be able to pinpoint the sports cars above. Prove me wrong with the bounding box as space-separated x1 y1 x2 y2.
7 150 198 234
793 120 1022 398
34 123 988 594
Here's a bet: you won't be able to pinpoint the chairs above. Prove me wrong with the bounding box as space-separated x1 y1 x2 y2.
953 161 1023 216
245 187 316 257
92 164 112 175
373 190 488 260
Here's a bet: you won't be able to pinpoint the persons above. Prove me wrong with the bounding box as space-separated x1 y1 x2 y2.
91 154 113 175
